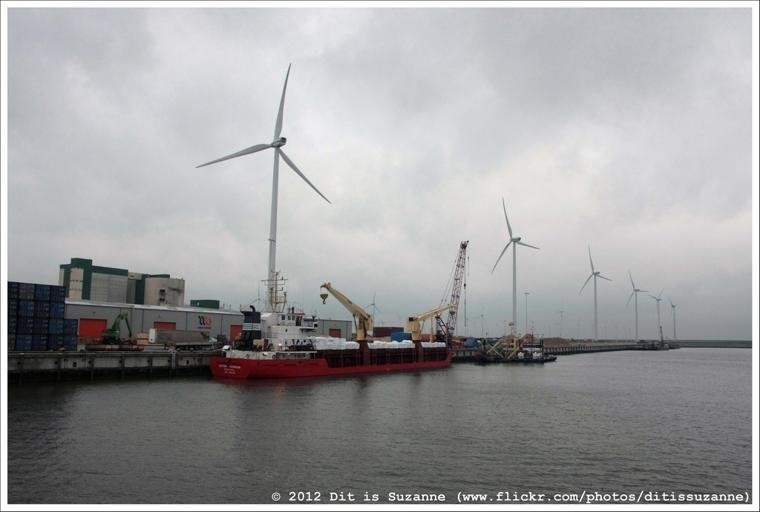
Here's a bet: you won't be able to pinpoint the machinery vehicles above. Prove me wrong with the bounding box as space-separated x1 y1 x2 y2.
101 308 133 343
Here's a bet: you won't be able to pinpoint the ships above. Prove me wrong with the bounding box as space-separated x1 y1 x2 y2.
210 268 458 381
